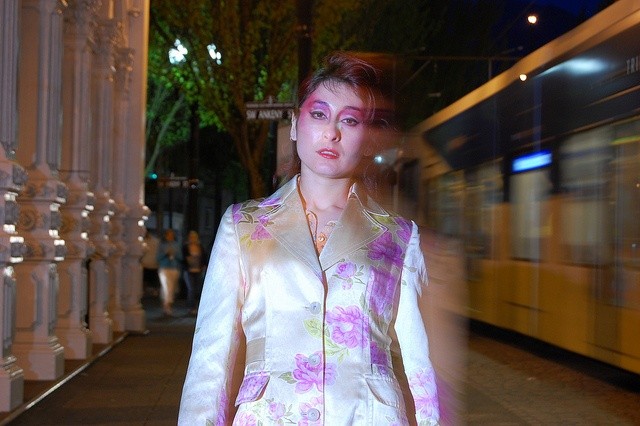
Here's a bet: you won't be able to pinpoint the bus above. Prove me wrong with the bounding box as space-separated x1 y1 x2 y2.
383 1 640 377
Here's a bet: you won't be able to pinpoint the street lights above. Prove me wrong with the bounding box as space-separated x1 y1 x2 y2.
168 34 223 237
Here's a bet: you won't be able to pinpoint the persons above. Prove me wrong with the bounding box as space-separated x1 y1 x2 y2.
142 228 162 299
176 58 445 426
182 230 209 316
156 229 183 319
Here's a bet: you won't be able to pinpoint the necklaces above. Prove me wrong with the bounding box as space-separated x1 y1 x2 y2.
304 209 336 256
